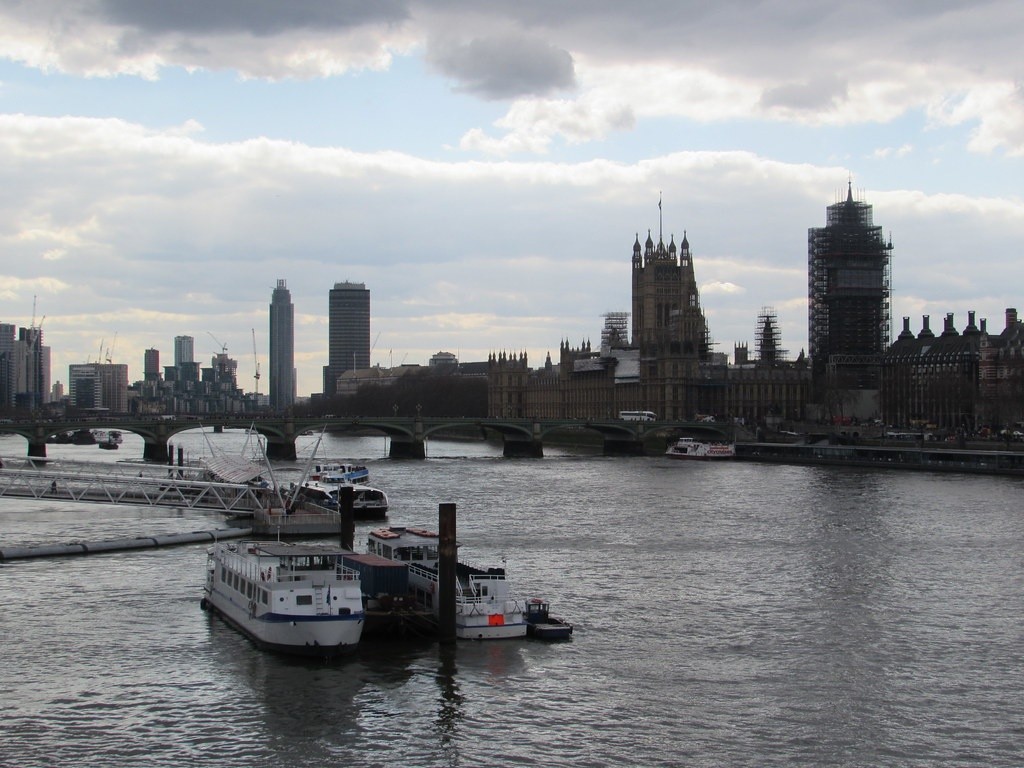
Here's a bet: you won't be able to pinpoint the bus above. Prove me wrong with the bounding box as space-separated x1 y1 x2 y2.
618 410 657 424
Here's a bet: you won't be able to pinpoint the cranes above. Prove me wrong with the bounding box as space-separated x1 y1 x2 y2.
207 332 229 354
83 329 119 365
24 297 46 355
252 328 261 401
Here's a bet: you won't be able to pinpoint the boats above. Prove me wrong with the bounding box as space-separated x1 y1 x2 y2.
200 522 366 662
309 457 373 490
666 437 737 460
520 596 575 640
369 522 528 638
291 472 389 519
